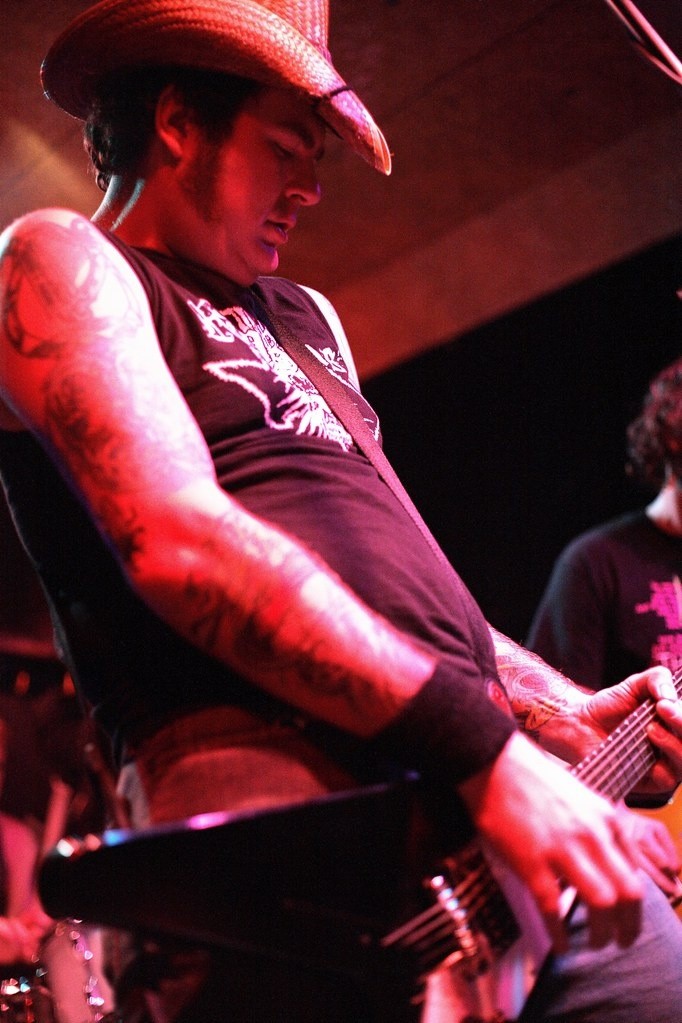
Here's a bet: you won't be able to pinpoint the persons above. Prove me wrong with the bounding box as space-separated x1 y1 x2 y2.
0 0 681 1023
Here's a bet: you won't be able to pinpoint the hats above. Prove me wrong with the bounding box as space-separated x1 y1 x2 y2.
40 0 392 176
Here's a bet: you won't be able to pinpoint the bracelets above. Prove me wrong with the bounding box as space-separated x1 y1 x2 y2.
363 667 519 791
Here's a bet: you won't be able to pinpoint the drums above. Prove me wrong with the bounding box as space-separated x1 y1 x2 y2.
35 916 138 1022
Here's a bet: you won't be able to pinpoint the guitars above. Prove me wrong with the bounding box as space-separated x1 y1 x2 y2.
30 666 682 1023
